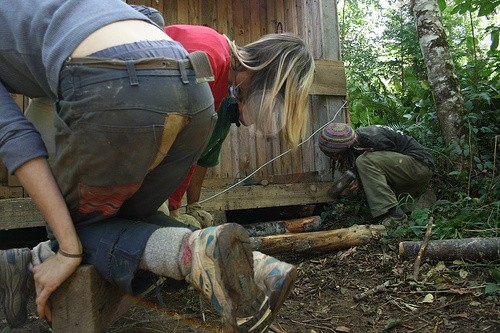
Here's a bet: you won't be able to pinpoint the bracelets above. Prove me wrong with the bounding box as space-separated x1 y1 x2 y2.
55 247 85 259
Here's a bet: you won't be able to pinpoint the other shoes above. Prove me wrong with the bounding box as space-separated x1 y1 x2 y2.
378 214 408 228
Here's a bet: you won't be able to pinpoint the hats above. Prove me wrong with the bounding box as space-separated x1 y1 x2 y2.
319 122 357 154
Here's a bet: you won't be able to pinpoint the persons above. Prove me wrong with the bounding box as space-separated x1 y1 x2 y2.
1 0 298 333
0 24 315 333
318 122 436 227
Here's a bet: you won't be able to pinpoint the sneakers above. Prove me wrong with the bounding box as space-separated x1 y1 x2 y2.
184 222 273 333
246 249 298 323
0 247 35 328
